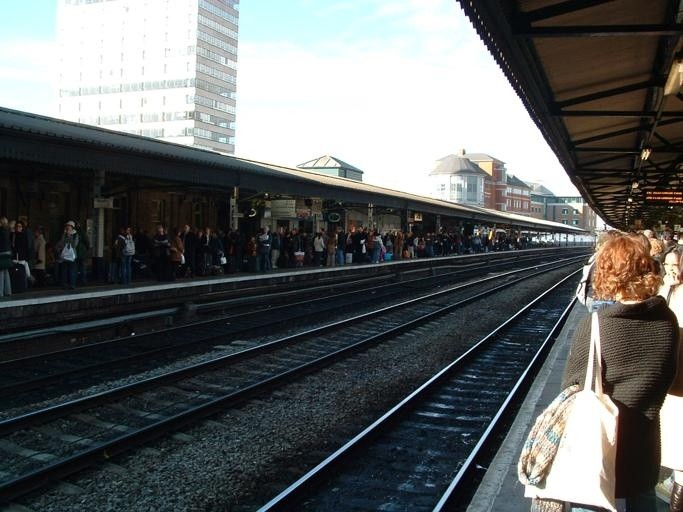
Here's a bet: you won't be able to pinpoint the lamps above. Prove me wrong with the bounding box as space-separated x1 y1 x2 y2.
638 52 683 162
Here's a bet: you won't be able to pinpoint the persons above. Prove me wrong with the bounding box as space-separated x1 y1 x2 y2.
562 225 683 512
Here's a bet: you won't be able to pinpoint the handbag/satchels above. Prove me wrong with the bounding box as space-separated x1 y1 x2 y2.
220 256 227 265
60 243 75 262
181 253 186 264
524 312 619 512
361 244 366 254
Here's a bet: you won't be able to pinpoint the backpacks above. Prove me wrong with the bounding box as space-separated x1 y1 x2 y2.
575 253 596 306
117 235 135 256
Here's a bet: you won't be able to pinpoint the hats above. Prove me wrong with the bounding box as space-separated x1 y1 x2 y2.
66 221 75 227
293 250 304 261
